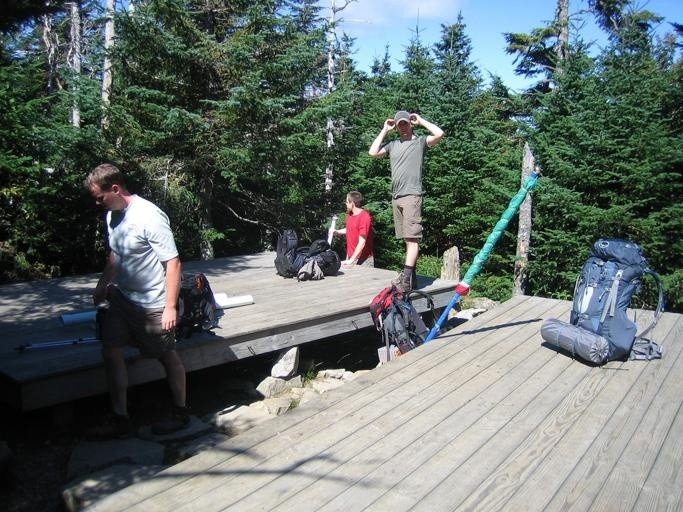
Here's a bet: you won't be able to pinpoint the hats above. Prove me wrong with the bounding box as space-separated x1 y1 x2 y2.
394 111 410 126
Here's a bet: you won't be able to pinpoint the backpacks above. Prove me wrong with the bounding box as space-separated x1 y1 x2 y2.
368 283 439 354
569 238 665 361
274 227 341 278
177 272 218 332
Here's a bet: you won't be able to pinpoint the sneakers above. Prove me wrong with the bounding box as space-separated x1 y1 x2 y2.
85 411 137 441
151 404 192 434
396 272 416 292
391 270 403 287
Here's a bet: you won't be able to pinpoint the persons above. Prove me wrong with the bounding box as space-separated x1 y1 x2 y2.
368 108 446 295
329 189 375 268
83 161 191 442
